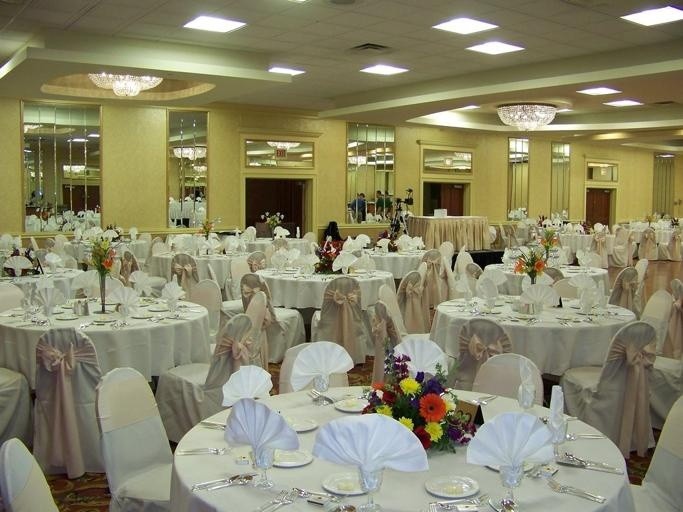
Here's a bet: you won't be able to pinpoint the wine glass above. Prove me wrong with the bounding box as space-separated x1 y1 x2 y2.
272 250 378 277
462 285 609 322
313 372 331 406
39 281 180 328
518 387 536 415
545 418 567 458
356 464 385 512
251 448 276 490
499 461 525 507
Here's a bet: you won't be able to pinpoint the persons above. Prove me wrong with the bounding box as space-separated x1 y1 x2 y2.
351 193 364 212
384 191 392 216
323 221 343 240
376 190 383 217
360 193 367 221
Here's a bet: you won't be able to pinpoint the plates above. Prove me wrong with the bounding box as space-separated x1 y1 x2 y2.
28 295 170 322
252 394 540 497
281 263 377 279
475 294 604 320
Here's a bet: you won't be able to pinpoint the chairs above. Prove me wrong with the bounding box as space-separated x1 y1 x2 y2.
168 197 207 228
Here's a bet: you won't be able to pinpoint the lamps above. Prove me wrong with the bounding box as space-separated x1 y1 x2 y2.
173 148 207 161
266 141 302 157
496 103 557 136
88 73 164 97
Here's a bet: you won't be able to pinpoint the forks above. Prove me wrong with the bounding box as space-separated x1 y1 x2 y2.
546 476 606 504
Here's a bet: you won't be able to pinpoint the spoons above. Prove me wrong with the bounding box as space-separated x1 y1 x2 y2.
192 474 243 488
208 475 253 491
